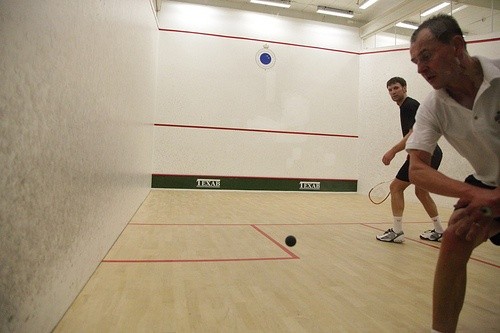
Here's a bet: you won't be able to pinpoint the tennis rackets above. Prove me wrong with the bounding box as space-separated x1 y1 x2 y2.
368 178 399 205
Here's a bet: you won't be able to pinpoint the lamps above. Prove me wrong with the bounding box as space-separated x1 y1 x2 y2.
317 0 377 19
250 0 291 9
394 2 468 35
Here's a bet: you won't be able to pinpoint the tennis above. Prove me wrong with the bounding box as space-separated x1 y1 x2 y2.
284 235 297 248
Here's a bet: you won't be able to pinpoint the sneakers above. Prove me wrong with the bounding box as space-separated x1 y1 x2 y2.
420 229 444 242
376 228 405 243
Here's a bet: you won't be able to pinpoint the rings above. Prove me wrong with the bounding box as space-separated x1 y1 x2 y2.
473 222 482 227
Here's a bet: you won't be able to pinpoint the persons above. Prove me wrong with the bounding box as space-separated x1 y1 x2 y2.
376 77 444 243
405 12 500 333
